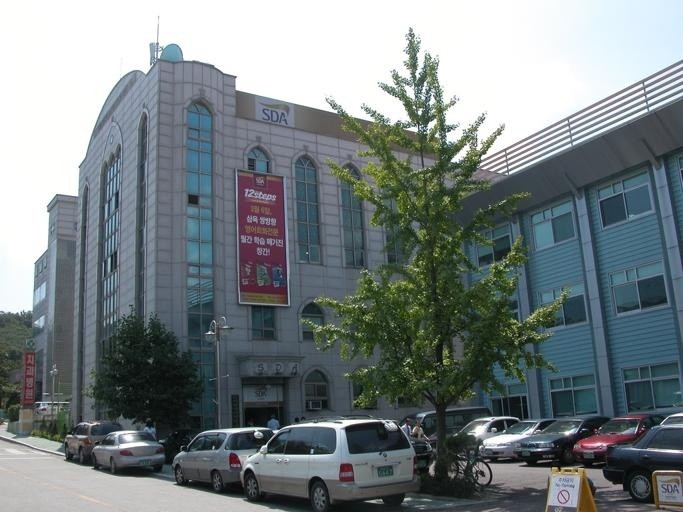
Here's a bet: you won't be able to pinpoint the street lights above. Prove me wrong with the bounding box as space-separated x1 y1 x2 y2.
203 310 234 429
47 360 57 422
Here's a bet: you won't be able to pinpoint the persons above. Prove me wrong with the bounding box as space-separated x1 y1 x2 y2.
144 419 156 440
247 416 256 427
411 419 430 441
401 417 411 440
267 414 280 429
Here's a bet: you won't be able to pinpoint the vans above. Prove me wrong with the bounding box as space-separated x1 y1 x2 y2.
397 405 494 452
170 425 276 494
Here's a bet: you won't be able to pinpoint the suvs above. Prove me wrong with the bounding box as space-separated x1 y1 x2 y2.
237 413 423 510
61 420 122 463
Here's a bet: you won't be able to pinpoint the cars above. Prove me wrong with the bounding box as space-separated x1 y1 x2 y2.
512 414 611 465
90 430 165 473
477 417 559 461
34 403 59 417
659 412 683 428
602 424 682 502
452 415 521 458
572 413 665 466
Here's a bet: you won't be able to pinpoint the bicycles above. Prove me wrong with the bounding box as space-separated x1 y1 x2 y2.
443 448 493 486
